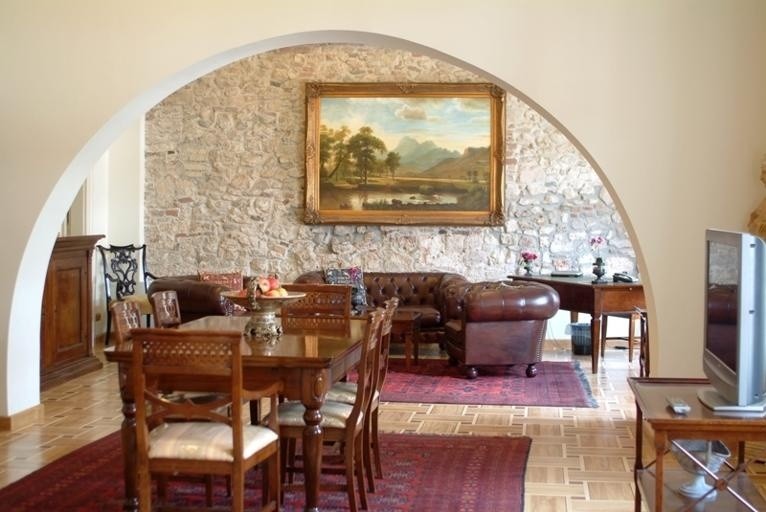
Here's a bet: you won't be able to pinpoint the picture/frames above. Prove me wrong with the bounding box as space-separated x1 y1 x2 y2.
305 81 505 226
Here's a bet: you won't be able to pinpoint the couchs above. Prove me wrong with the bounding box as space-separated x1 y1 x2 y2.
295 271 468 351
443 281 560 379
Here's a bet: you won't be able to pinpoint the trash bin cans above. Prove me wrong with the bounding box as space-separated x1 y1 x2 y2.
570 322 592 356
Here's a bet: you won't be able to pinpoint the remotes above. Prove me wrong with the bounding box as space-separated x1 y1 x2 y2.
664 395 691 414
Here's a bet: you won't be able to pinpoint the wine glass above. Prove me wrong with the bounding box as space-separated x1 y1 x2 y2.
672 437 732 500
219 288 308 339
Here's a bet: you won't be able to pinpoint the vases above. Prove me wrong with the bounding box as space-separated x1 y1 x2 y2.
524 260 532 276
593 258 608 283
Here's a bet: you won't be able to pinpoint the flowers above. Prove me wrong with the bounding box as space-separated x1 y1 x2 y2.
522 252 536 261
591 238 605 256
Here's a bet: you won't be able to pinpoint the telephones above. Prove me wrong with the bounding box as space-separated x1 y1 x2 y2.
613 273 632 282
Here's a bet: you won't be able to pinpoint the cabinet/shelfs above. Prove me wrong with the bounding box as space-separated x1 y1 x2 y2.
40 235 105 391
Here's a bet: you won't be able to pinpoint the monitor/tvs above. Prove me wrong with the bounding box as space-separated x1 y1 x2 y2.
695 228 766 412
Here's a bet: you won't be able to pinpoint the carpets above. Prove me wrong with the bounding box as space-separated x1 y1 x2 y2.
0 430 532 512
347 359 600 408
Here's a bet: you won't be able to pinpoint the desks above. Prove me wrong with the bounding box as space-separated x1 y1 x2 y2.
627 377 766 512
507 276 647 374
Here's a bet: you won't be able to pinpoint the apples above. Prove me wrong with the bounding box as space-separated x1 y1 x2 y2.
239 274 288 297
